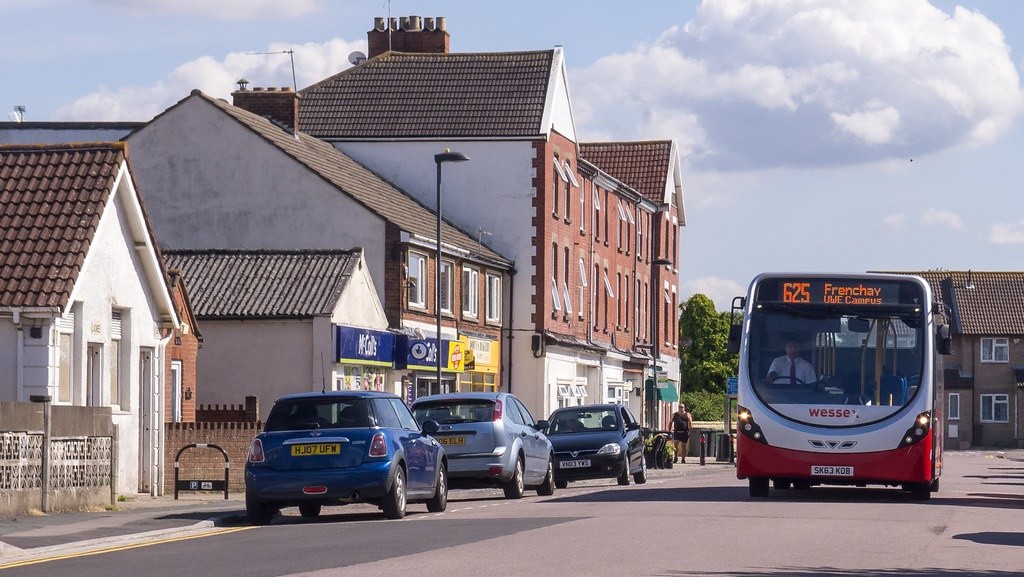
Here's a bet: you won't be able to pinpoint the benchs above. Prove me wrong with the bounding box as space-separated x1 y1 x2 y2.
644 434 668 469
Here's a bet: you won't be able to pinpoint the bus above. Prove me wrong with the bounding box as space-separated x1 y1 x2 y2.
727 272 943 501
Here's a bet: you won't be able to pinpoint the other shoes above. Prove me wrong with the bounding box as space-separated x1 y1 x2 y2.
673 458 678 463
682 460 685 463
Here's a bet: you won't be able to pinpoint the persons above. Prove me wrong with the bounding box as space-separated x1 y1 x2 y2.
668 403 692 464
765 339 817 384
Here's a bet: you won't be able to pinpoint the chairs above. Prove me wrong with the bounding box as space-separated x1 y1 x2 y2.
338 405 366 427
566 415 617 431
879 367 920 404
430 406 491 424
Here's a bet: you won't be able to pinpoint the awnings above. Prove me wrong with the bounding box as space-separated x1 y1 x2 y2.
646 378 678 403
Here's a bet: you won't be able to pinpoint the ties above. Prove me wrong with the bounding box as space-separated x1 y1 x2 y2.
790 360 796 385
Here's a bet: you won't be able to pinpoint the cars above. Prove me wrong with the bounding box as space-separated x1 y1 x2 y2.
542 403 648 488
410 392 555 499
245 392 447 523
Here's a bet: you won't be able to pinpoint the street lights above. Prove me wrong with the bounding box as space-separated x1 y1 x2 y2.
434 148 469 388
